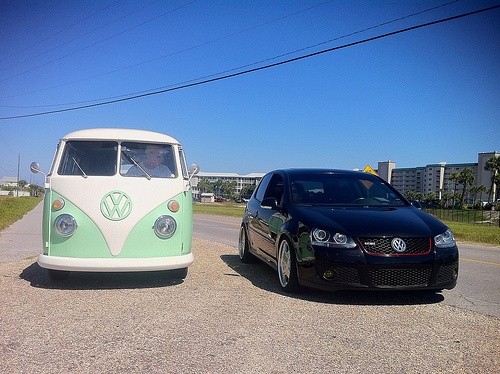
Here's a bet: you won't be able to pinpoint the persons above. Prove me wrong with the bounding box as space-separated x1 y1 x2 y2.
126 145 173 177
292 183 312 204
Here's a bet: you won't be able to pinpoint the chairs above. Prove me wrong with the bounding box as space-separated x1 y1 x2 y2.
280 183 306 206
339 179 368 203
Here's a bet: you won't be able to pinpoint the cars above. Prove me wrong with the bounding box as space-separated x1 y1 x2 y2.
238 169 459 295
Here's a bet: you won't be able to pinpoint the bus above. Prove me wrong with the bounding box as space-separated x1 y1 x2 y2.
29 128 200 280
